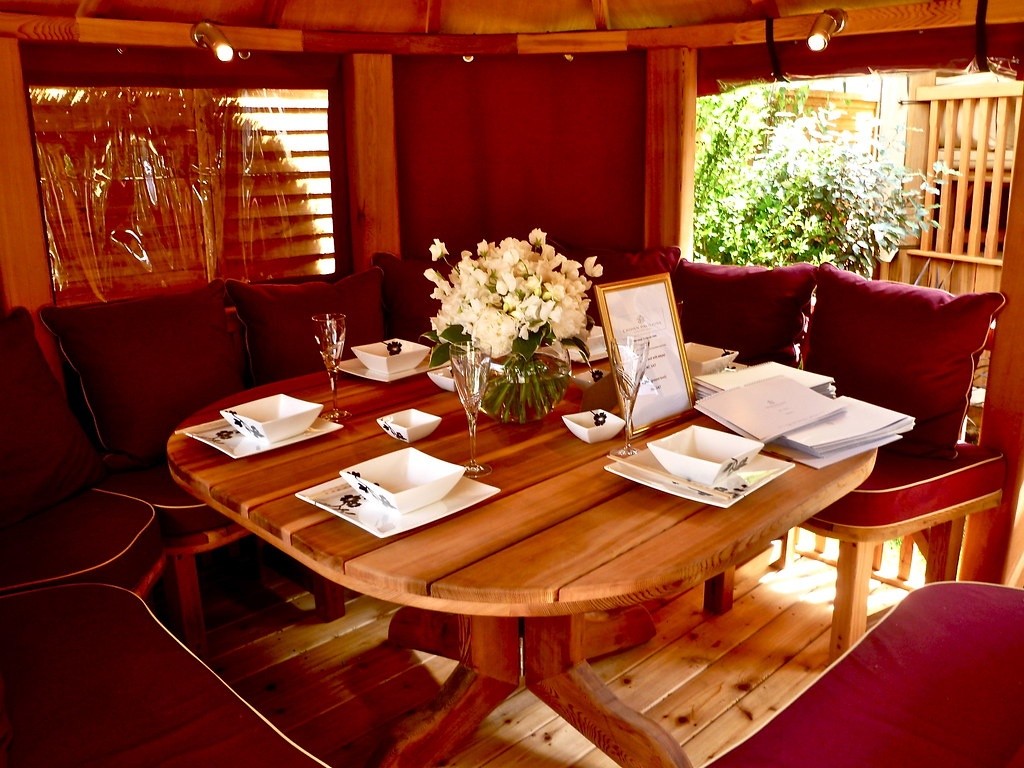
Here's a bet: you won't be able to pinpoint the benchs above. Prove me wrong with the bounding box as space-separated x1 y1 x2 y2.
4 441 1023 767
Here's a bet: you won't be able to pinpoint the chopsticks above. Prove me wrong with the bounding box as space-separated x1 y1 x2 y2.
606 453 734 501
174 420 230 434
305 482 350 502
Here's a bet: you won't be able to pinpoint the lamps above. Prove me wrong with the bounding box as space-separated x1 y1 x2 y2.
805 7 847 51
190 18 234 62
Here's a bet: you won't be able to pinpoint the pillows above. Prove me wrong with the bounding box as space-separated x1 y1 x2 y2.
798 262 996 467
226 260 385 388
557 245 680 323
369 252 460 347
1 306 101 541
671 256 817 374
38 275 245 471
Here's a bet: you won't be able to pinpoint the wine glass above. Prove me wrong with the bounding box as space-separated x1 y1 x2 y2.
448 341 492 478
311 313 352 420
610 335 650 457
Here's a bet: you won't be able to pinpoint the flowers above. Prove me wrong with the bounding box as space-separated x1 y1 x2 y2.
419 224 604 420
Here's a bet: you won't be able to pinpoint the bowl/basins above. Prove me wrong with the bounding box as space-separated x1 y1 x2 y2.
350 338 431 373
562 409 626 445
339 447 466 514
376 408 442 443
684 342 739 377
220 393 325 445
646 423 764 486
426 366 456 392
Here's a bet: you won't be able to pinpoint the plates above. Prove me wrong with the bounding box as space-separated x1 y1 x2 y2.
603 448 796 508
183 419 344 458
339 359 451 382
294 476 501 536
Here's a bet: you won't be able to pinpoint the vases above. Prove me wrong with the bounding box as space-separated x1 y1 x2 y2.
469 334 571 424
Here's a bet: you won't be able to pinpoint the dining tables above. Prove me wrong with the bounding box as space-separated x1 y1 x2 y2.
165 342 879 765
